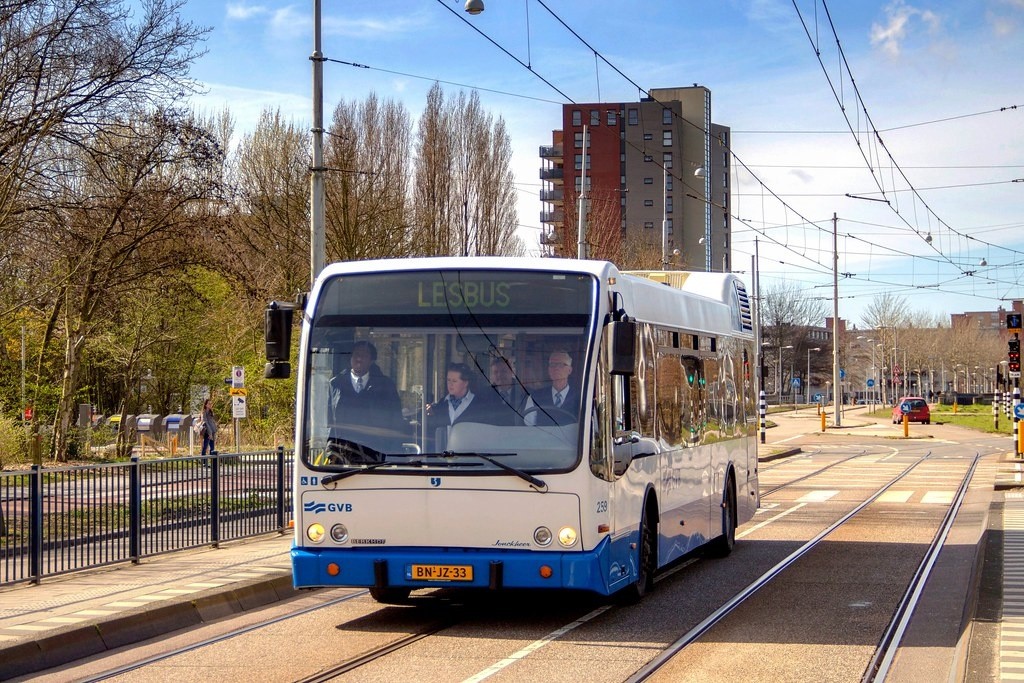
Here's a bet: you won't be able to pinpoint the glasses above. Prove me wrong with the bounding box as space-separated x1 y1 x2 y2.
549 362 568 369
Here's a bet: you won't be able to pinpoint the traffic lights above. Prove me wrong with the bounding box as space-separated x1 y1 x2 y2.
1006 313 1023 330
1008 341 1020 373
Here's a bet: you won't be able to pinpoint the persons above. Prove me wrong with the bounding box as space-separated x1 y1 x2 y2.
199 398 216 467
537 352 581 425
481 357 533 427
420 362 493 444
329 341 414 443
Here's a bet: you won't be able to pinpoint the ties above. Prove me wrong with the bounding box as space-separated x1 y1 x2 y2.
356 377 362 394
555 393 561 407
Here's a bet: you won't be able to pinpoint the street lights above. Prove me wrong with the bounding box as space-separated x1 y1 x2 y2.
779 346 794 407
856 335 993 397
875 325 899 404
806 348 821 406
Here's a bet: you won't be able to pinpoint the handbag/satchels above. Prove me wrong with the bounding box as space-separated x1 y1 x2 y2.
194 422 205 436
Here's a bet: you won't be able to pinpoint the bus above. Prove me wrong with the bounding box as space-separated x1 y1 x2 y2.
263 260 759 606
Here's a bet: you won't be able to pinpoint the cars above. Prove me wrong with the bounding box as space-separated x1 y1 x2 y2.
891 397 930 424
